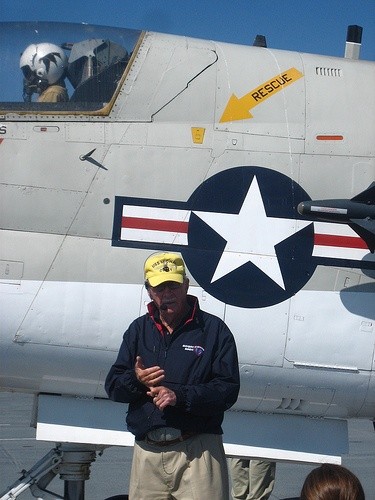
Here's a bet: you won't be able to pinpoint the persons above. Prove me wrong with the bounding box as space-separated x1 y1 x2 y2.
102 250 240 500
230 458 276 500
300 463 365 500
17 42 69 103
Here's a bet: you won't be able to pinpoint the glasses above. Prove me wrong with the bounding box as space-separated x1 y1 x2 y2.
154 281 180 292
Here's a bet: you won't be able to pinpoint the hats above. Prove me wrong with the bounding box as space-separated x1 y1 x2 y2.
144 253 185 287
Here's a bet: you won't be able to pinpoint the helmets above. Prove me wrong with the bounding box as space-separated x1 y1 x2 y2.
19 43 68 85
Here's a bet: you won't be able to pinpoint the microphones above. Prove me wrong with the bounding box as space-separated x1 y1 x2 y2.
152 298 167 310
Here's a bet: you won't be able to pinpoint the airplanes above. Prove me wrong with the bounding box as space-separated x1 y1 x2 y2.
0 20 374 500
